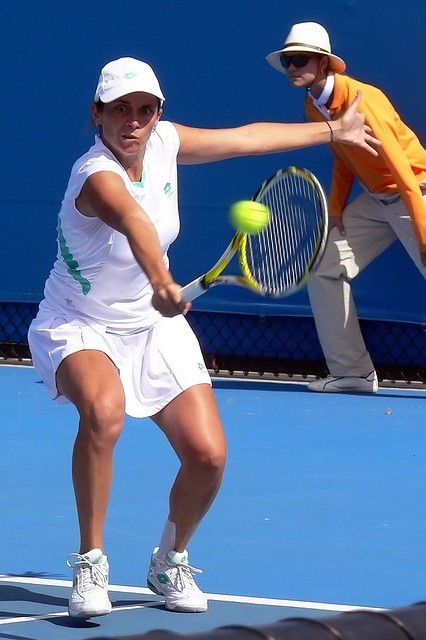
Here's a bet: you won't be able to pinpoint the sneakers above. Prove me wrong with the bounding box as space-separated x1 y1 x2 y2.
67 548 111 617
147 546 208 612
308 370 379 393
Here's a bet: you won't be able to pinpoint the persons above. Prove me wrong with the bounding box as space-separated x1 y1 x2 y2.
28 57 382 617
267 21 425 392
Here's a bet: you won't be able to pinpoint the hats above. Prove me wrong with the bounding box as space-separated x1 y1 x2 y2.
93 57 165 107
266 23 347 94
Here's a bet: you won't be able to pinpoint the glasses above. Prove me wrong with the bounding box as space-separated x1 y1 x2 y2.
280 55 321 68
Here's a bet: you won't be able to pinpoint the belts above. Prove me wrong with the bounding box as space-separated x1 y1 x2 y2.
380 187 426 205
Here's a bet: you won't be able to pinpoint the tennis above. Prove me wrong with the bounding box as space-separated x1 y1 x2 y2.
230 200 270 235
